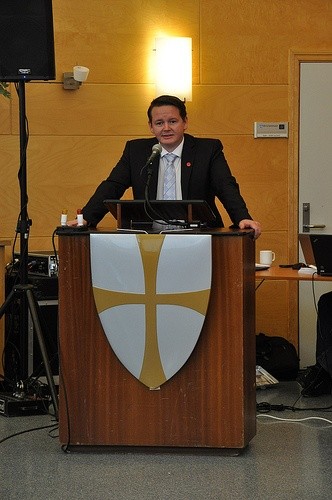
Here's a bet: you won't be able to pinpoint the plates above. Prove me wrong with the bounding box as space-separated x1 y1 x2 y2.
255 264 270 270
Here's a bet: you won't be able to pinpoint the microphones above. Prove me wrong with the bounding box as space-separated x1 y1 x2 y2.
145 143 162 168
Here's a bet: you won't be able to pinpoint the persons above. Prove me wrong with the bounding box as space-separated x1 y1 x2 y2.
66 96 262 240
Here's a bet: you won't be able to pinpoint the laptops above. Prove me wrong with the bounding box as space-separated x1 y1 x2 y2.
298 233 332 277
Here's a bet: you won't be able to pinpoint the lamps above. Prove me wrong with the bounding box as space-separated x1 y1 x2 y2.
151 38 194 103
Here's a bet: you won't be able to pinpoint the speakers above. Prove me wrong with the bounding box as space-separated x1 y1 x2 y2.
0 0 56 83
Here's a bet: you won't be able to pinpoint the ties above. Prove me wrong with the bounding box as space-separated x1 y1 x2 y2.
162 152 177 229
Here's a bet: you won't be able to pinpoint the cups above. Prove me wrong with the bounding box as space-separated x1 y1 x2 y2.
260 250 275 266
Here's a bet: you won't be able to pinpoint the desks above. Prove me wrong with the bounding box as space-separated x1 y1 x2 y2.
255 265 332 282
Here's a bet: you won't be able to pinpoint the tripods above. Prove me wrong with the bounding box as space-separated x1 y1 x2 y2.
0 82 59 423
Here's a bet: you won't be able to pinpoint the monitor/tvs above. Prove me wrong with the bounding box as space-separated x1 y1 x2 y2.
103 200 216 222
